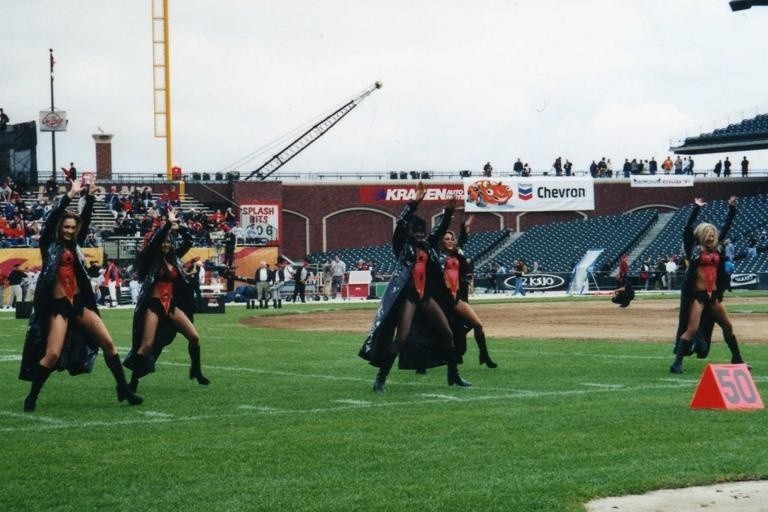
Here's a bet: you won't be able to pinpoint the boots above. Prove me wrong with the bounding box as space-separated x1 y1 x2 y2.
374 340 401 392
104 356 145 404
727 335 754 369
671 339 692 373
449 364 472 387
25 363 52 413
188 346 210 384
475 333 499 368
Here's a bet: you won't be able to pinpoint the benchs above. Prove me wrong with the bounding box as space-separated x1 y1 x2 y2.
0 192 225 259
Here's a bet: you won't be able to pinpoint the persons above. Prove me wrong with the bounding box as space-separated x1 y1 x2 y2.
484 260 543 296
0 162 258 247
415 213 498 374
8 255 373 308
669 196 752 374
18 174 143 412
483 155 749 177
609 235 757 308
358 182 472 393
123 207 211 393
0 108 9 130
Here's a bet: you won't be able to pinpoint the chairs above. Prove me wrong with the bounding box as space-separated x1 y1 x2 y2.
306 195 768 287
679 102 767 143
